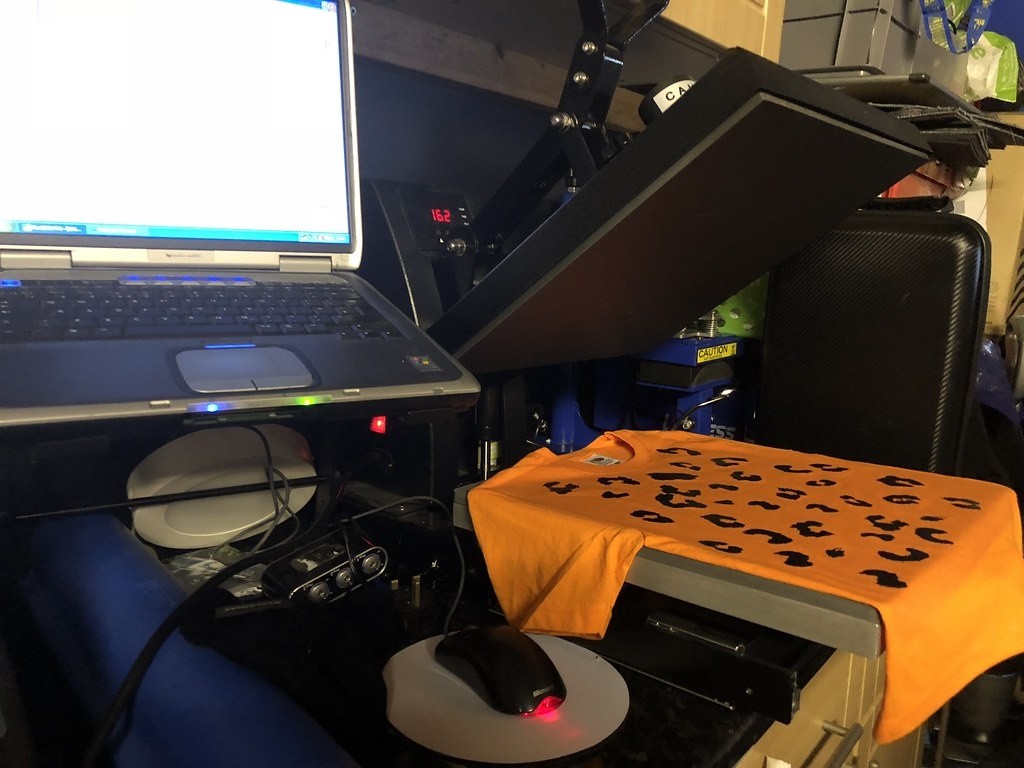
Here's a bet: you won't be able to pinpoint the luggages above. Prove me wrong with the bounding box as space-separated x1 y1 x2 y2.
754 194 992 482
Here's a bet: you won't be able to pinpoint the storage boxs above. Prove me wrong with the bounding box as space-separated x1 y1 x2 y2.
658 1 971 198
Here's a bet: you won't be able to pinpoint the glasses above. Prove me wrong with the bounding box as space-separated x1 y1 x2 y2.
912 170 968 202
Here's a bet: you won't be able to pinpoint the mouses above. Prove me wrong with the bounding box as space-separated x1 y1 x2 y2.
434 624 568 717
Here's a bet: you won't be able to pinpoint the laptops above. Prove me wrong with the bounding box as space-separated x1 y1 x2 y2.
0 0 482 448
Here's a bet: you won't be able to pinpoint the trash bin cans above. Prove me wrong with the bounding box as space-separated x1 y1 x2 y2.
954 658 1019 746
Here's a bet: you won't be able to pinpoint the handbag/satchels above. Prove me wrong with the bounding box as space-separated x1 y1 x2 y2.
912 0 1017 102
